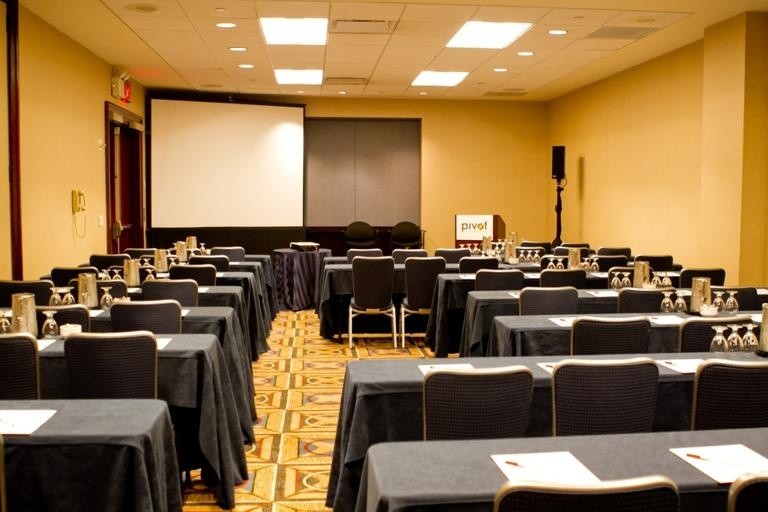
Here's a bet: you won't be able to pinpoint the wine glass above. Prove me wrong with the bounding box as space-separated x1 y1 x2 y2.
709 323 760 351
459 237 738 314
0 240 207 339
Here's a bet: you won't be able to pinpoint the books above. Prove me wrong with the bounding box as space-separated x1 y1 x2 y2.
647 314 684 326
156 337 172 351
586 290 619 298
736 313 763 322
36 339 57 351
490 450 601 487
668 443 768 486
548 316 586 328
653 357 706 375
0 409 57 435
417 363 476 377
536 361 558 373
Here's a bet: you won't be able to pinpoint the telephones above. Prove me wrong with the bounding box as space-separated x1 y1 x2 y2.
72 189 87 214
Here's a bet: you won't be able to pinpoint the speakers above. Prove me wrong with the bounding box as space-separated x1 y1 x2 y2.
552 146 565 179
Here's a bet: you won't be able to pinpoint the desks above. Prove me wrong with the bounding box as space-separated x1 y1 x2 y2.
273 248 332 311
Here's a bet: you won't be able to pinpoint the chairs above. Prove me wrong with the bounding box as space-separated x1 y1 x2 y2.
317 239 768 510
0 246 272 511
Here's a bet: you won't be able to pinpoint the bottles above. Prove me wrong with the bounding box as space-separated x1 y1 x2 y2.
757 303 768 354
